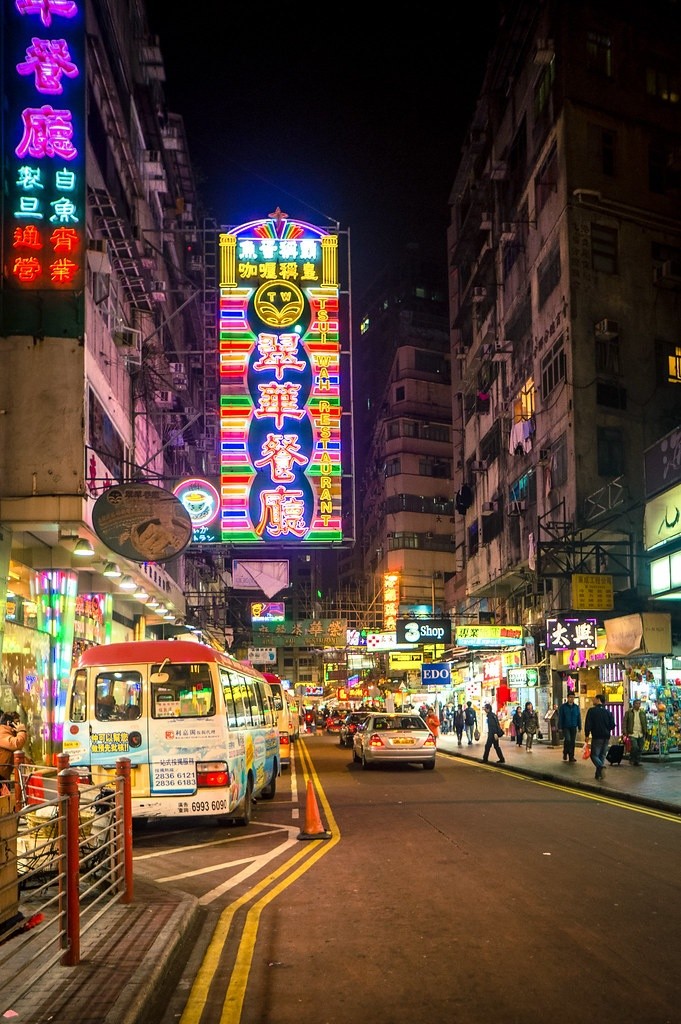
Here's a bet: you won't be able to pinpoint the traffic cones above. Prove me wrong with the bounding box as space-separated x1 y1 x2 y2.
297 779 334 841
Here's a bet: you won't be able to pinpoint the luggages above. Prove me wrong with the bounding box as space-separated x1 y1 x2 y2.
605 734 628 766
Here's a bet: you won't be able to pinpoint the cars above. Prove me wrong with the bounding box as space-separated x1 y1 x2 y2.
339 712 369 747
352 713 437 769
327 708 353 733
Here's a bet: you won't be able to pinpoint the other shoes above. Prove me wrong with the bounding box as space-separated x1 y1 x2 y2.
595 766 606 780
0 911 42 944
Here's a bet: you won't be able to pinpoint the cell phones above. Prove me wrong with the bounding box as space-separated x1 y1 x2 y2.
9 721 16 726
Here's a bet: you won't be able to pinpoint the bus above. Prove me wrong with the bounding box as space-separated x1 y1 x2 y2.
62 640 283 829
261 672 293 770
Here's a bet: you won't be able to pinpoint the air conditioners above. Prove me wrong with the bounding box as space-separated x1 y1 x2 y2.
489 341 512 362
482 501 498 516
594 319 618 340
151 279 167 302
174 375 188 391
169 362 187 380
531 38 554 65
507 501 524 516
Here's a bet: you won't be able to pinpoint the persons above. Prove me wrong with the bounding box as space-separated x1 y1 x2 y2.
299 693 648 781
0 694 139 791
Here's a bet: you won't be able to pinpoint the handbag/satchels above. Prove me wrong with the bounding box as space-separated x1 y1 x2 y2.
538 731 543 739
474 729 480 741
496 729 505 737
581 741 591 760
559 729 565 740
